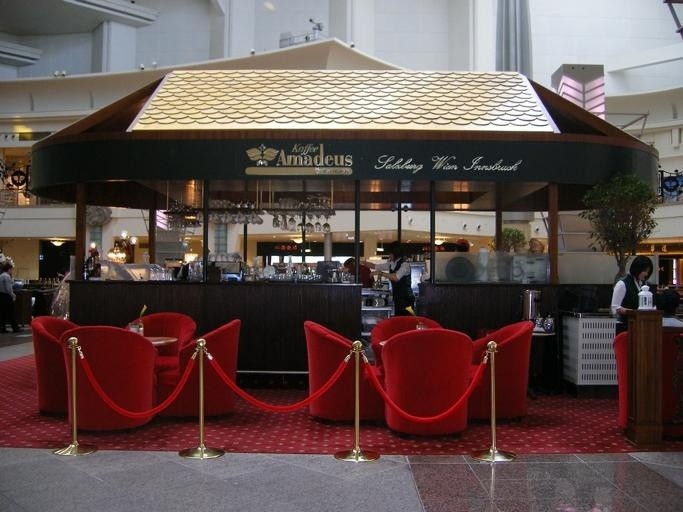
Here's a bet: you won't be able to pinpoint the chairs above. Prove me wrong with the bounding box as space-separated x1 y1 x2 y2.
303 317 535 436
31 310 242 435
612 328 683 430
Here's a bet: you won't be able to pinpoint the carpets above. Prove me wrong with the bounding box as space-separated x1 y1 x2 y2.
1 353 681 455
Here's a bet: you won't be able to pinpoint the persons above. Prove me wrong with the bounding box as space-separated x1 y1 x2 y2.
607 253 653 338
359 239 416 317
0 260 24 334
343 258 376 307
654 287 683 328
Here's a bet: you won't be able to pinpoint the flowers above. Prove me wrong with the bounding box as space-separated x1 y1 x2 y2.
139 304 147 320
405 306 419 323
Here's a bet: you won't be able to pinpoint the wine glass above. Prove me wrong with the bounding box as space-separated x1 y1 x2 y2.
272 213 332 234
